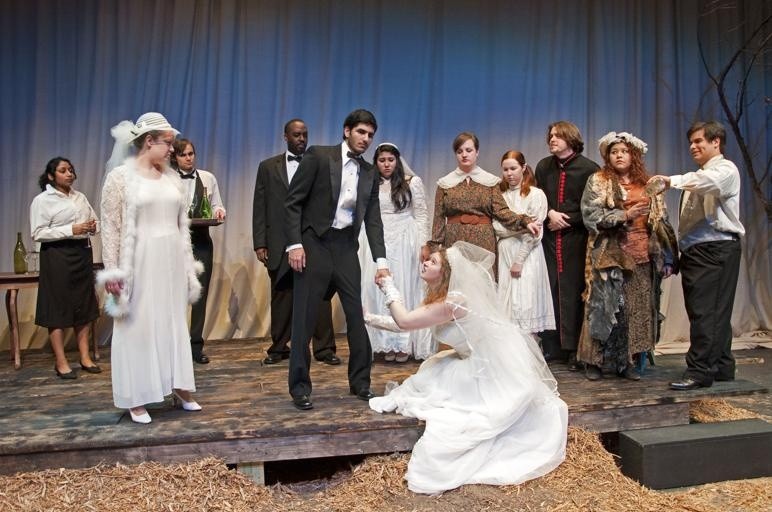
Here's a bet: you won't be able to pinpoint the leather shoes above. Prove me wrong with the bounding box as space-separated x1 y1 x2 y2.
128 404 152 424
192 349 209 364
713 369 735 381
293 391 313 410
350 388 376 400
317 352 341 365
567 352 582 371
384 351 409 362
264 351 290 365
616 367 642 380
585 362 601 380
668 375 712 390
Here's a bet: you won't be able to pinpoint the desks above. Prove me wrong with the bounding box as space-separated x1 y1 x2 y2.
1 262 104 371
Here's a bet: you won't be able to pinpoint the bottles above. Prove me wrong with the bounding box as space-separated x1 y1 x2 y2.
638 178 665 199
13 231 27 274
200 186 214 218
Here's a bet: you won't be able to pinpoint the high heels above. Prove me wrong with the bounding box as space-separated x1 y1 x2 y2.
79 358 102 375
54 361 78 380
171 387 202 411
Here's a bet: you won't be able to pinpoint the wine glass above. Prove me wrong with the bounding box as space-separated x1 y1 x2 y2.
30 244 40 274
84 218 95 249
187 192 198 219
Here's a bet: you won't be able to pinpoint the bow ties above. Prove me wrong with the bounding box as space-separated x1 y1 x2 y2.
347 151 362 164
287 155 302 163
177 168 196 180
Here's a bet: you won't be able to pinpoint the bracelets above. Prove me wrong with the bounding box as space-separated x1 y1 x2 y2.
626 210 630 221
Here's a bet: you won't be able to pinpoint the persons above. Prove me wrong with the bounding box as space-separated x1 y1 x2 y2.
432 132 537 291
577 132 667 381
99 112 205 425
645 118 745 391
533 120 600 372
364 246 540 415
167 139 227 364
355 142 427 364
280 108 390 410
491 149 557 337
29 157 104 380
251 117 344 366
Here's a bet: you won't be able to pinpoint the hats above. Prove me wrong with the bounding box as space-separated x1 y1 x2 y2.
128 111 181 148
598 129 648 162
